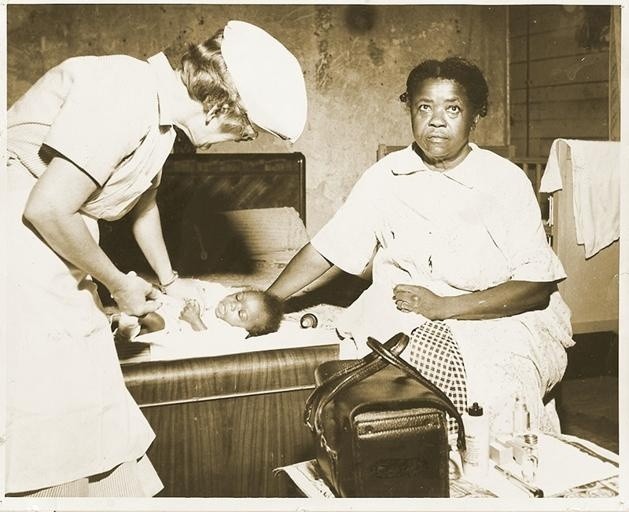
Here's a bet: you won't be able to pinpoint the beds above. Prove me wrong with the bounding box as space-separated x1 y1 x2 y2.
376 142 621 336
90 152 356 497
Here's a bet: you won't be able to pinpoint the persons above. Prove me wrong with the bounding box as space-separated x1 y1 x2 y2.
107 288 282 348
264 54 577 433
7 17 308 495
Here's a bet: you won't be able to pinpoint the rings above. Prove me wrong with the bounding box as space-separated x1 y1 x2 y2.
413 295 420 302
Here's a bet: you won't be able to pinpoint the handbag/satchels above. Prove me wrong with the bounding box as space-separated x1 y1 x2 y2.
302 330 466 498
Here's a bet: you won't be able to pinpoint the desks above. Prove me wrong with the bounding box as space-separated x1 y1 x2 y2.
285 426 619 497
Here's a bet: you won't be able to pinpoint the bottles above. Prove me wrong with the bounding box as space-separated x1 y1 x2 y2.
461 402 490 466
512 401 530 433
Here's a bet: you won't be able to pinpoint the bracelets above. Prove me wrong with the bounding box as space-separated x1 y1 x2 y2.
161 269 179 288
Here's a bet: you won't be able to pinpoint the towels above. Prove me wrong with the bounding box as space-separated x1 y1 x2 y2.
538 137 620 259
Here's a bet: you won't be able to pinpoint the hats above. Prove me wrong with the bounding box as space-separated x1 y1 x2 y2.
219 19 308 146
